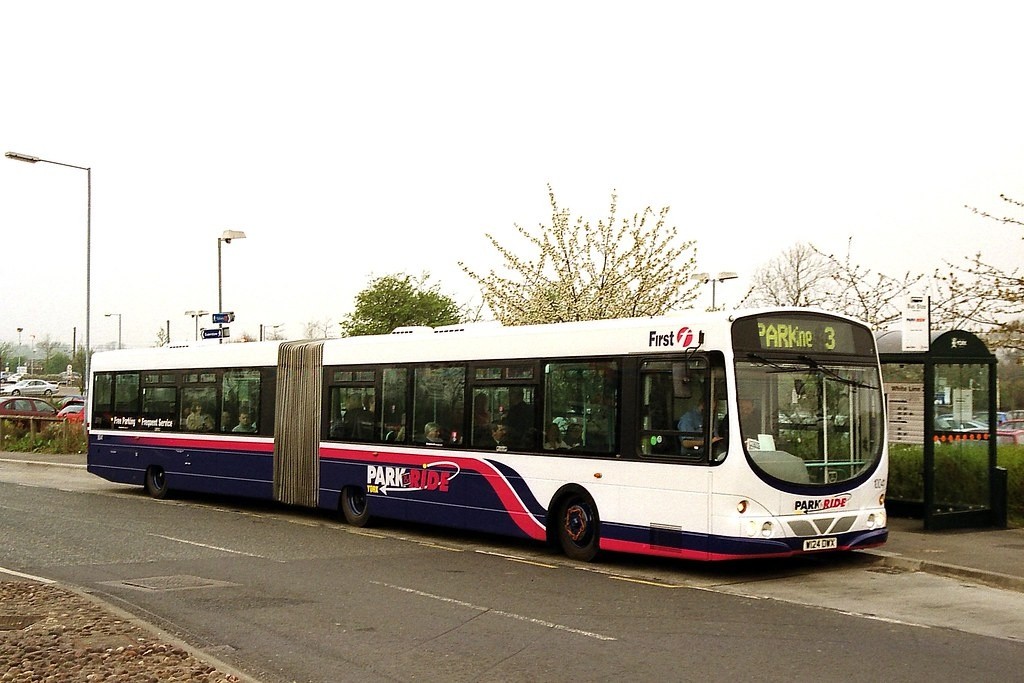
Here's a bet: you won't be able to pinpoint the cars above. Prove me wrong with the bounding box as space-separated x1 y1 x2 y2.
935 409 1024 445
717 411 849 441
0 372 84 424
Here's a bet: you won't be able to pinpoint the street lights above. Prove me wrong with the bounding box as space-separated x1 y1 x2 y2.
691 272 739 312
6 152 91 426
264 326 279 342
218 230 246 344
17 328 24 373
105 313 121 349
30 335 35 374
185 311 209 342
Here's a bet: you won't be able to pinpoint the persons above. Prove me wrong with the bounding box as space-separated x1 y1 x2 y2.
421 422 445 443
383 411 416 443
463 387 536 450
718 399 761 450
220 401 258 432
330 393 375 440
544 422 583 454
181 396 216 431
677 395 724 456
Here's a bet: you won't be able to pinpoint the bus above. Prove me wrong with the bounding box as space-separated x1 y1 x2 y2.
86 306 889 563
